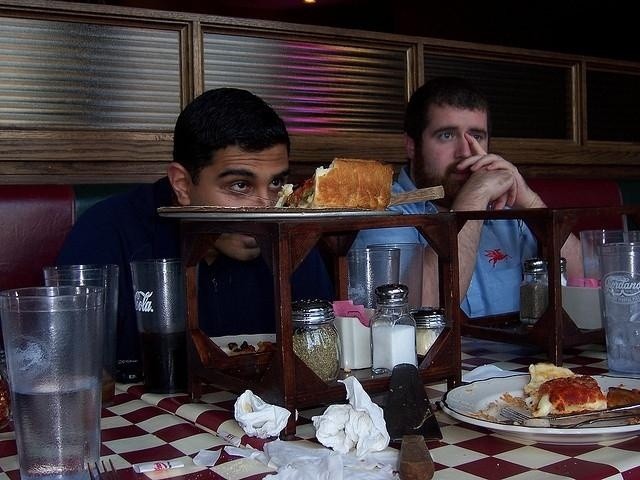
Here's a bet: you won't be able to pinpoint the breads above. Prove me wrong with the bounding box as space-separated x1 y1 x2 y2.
523 362 640 418
275 157 394 211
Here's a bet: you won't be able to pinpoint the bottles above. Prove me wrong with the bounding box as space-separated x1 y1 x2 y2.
290 298 341 383
372 284 445 373
519 258 567 326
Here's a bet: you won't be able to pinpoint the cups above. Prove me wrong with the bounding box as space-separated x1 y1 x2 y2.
129 257 188 393
347 243 424 307
580 230 639 376
0 285 104 480
43 265 117 409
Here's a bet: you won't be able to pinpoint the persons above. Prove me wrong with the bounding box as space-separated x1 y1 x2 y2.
347 79 602 318
41 89 337 371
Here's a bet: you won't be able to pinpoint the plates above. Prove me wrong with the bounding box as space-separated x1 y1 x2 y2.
441 375 640 445
207 332 277 359
